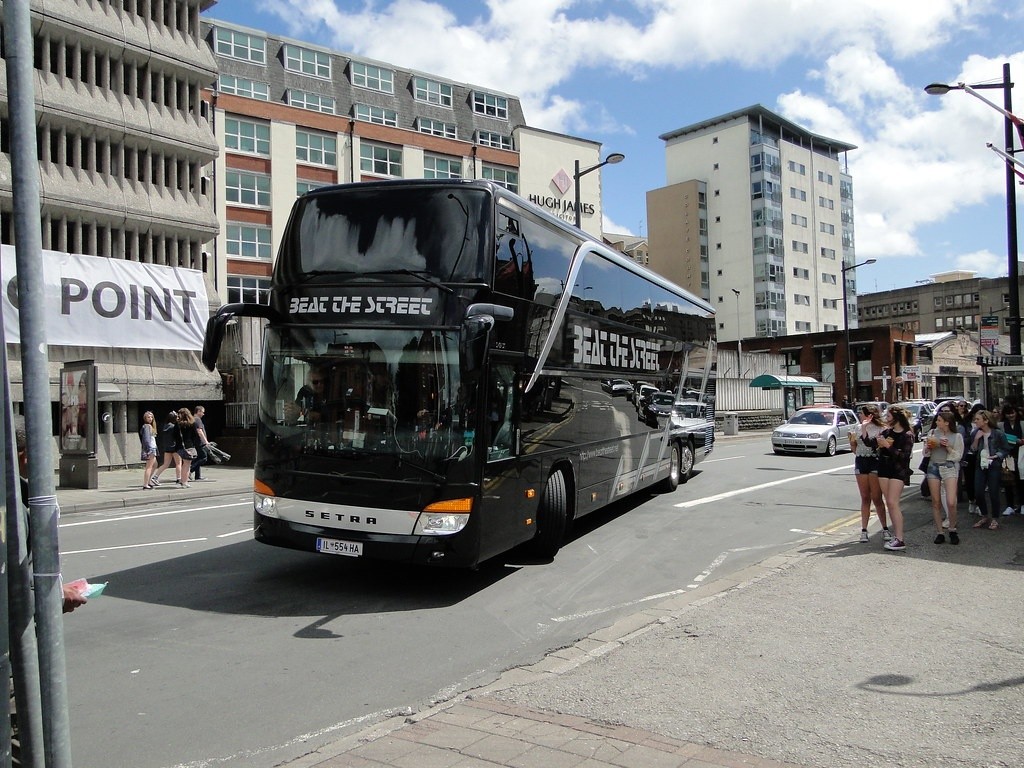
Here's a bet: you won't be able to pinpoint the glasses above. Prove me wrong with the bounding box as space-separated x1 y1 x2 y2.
310 380 324 385
959 405 967 407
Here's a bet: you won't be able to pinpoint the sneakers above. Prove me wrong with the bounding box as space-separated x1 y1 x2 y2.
860 532 868 541
881 530 891 541
884 537 906 550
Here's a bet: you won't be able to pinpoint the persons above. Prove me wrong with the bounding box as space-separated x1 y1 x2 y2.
151 412 183 485
12 413 87 614
920 400 1024 530
188 406 209 481
876 405 914 550
282 366 341 424
847 405 890 542
922 412 964 546
841 395 861 409
172 408 201 489
139 410 158 490
62 372 87 450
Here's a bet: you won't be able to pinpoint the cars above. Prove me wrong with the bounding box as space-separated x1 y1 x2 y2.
772 396 1003 456
601 377 714 445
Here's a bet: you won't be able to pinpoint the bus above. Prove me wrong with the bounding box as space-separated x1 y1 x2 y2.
201 178 718 570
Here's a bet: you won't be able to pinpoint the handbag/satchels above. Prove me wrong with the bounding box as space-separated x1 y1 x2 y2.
183 447 197 459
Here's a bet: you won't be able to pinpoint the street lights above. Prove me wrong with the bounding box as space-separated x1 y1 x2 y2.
989 306 1009 355
573 153 625 230
924 63 1021 357
333 331 348 344
841 257 877 410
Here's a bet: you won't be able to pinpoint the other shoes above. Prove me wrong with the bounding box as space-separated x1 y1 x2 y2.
949 531 959 544
976 506 984 516
942 518 956 528
969 499 976 513
176 480 182 484
1002 507 1015 515
934 534 945 543
195 477 207 481
989 520 998 529
188 473 192 480
151 478 162 486
143 486 152 490
182 484 190 488
